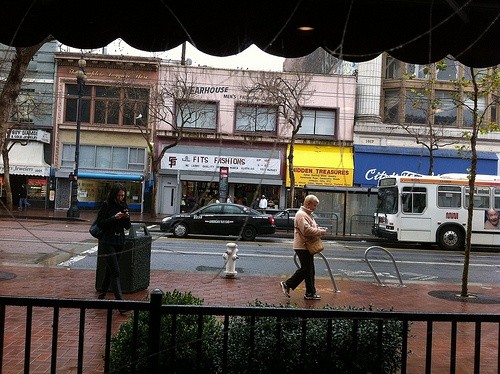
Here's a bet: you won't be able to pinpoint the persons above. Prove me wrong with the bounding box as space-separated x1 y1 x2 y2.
259 195 267 209
280 195 327 300
96 182 132 314
484 210 500 230
227 195 233 203
254 196 259 210
216 197 221 203
18 182 27 208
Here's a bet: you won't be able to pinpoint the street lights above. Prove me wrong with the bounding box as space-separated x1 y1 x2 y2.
67 56 88 220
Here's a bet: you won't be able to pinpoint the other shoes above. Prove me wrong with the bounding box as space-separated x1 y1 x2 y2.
280 281 290 298
304 293 320 300
98 294 109 300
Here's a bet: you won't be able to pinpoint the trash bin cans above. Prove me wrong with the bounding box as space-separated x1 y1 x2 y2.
95 223 152 293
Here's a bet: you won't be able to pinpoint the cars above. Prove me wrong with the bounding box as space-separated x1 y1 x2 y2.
159 202 276 239
267 207 333 229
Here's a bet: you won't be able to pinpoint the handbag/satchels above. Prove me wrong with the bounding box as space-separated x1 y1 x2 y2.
88 220 107 240
305 236 324 255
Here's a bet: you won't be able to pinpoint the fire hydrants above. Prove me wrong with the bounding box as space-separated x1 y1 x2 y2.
223 243 240 278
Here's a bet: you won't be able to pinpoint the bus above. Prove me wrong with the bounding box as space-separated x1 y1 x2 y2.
372 170 500 249
